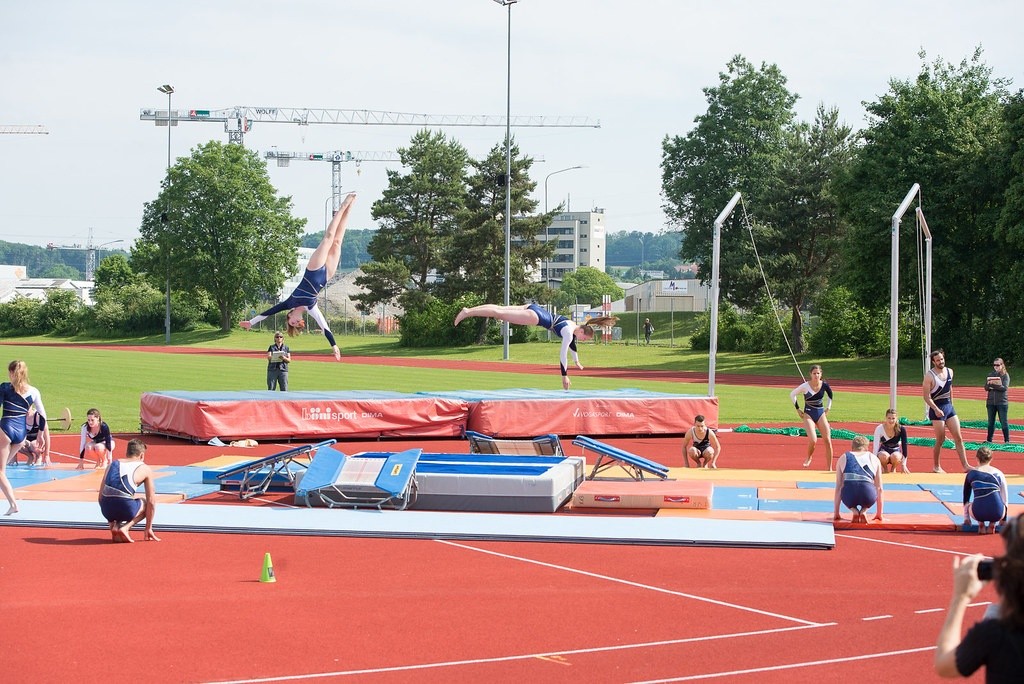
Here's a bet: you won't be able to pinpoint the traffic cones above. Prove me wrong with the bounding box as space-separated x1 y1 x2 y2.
258 552 278 583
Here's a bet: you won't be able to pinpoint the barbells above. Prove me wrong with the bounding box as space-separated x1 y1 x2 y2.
46 407 75 431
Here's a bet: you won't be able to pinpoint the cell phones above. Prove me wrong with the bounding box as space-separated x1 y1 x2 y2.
977 559 993 580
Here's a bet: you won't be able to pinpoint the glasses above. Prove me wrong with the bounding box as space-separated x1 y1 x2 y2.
275 336 282 338
994 364 1000 366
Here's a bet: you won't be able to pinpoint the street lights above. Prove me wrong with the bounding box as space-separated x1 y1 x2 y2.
156 81 176 339
98 239 124 276
495 0 521 360
543 164 588 346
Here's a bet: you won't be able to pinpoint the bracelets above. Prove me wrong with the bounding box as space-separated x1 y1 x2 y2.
45 454 49 456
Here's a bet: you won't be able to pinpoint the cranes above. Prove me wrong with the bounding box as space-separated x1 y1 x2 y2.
138 102 604 157
45 227 138 283
266 145 420 287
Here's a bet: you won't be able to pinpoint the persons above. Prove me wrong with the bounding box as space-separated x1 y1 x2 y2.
643 318 652 343
0 360 46 515
267 331 291 391
791 365 834 471
8 405 52 467
932 491 1024 684
239 194 357 362
984 358 1010 443
682 415 721 468
833 436 884 523
75 409 116 469
922 350 975 473
873 409 911 474
455 304 620 390
963 446 1008 534
99 439 161 543
